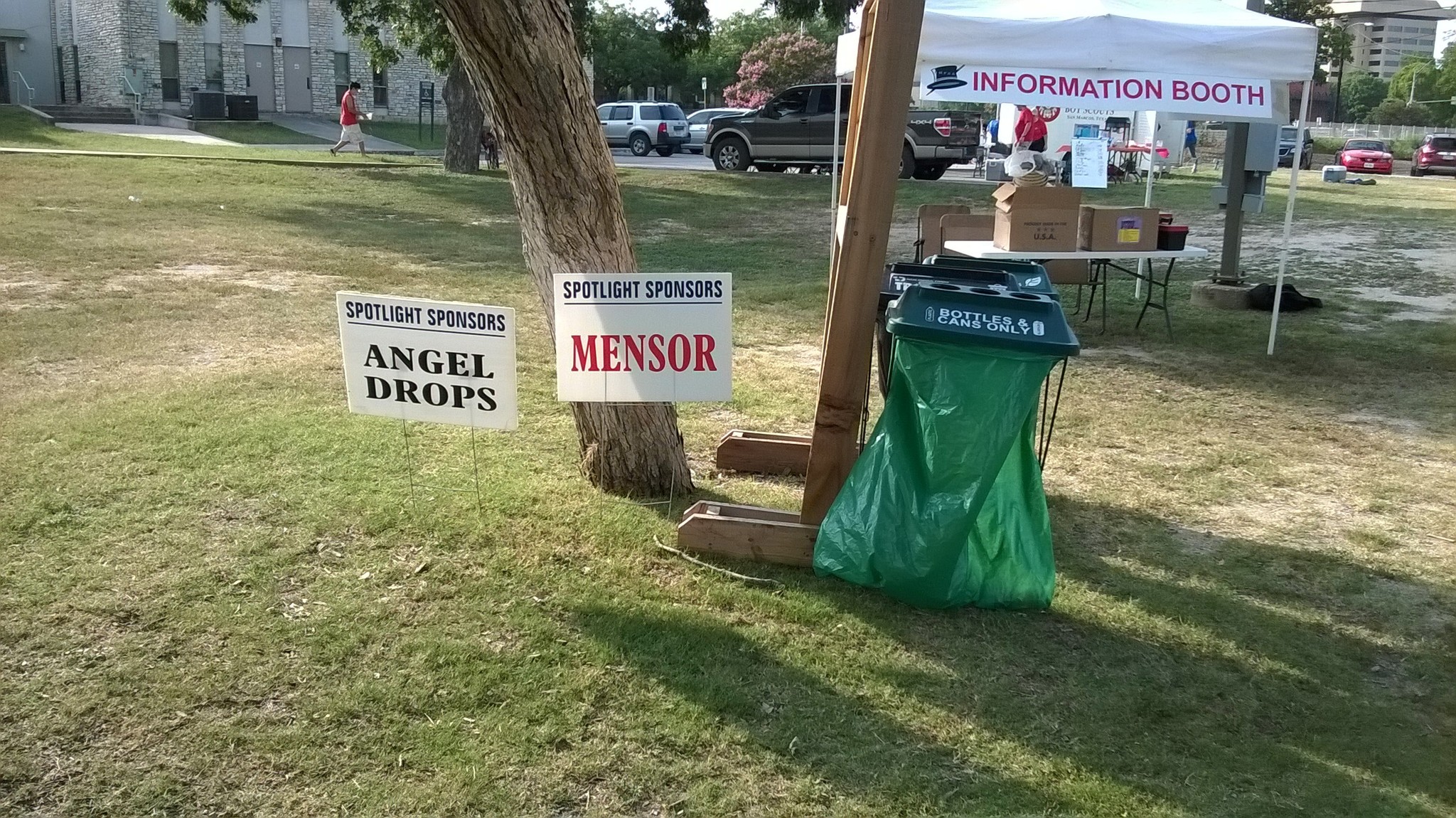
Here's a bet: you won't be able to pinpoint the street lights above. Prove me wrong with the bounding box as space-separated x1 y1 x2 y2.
1310 20 1375 123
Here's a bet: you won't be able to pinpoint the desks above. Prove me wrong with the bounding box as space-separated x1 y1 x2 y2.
1056 143 1168 185
944 240 1208 339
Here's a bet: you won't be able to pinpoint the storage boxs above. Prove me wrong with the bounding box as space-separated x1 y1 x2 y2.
1322 165 1347 182
986 153 1013 181
992 181 1081 252
1078 204 1161 252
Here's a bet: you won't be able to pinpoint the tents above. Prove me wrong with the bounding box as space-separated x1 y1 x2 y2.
836 0 1320 356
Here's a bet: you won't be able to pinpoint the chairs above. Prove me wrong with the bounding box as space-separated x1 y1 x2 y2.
1043 259 1106 334
940 212 994 257
914 204 970 263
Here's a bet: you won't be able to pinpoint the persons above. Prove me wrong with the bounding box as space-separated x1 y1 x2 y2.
1182 120 1199 170
1011 104 1048 154
329 82 371 159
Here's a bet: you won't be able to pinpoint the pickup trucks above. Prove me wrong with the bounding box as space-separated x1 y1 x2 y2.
703 83 982 181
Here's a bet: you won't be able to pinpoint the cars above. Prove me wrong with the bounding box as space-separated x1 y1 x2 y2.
673 107 752 154
1334 139 1394 175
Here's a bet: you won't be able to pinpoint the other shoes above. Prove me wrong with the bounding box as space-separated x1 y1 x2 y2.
330 148 336 158
1191 167 1196 173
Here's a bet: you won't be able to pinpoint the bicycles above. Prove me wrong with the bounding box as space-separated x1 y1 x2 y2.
481 130 499 170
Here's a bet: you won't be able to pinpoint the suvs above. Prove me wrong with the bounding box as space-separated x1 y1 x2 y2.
1411 132 1456 179
1277 125 1314 170
595 100 691 157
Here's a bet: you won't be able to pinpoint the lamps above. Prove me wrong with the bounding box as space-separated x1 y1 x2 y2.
19 44 25 51
275 37 282 47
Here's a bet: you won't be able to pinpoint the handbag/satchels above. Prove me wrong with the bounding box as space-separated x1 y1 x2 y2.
1247 283 1323 314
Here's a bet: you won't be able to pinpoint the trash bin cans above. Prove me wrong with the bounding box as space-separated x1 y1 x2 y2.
812 253 1082 616
